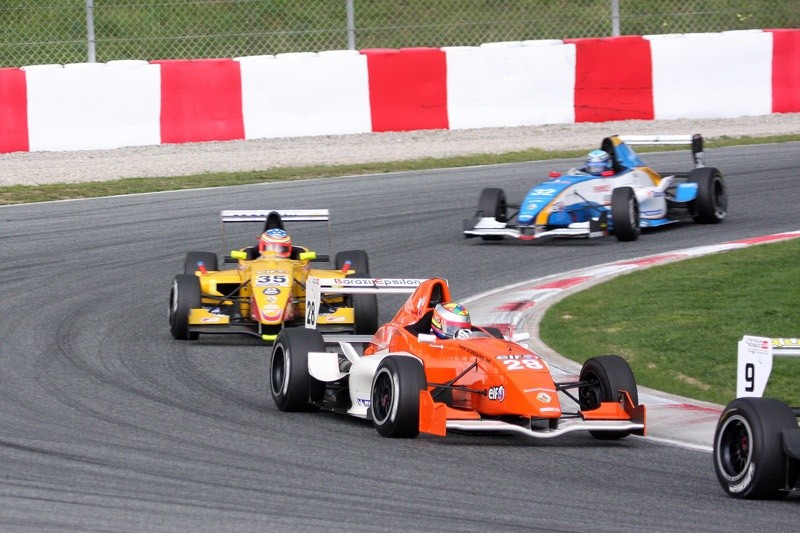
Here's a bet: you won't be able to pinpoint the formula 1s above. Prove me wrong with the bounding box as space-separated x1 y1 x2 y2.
166 207 379 343
462 133 729 245
268 274 646 442
710 334 800 504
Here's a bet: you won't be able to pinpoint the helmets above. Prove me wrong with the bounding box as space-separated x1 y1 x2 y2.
431 303 471 339
258 229 293 259
584 150 612 176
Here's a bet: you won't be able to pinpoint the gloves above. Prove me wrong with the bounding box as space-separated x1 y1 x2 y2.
453 328 472 340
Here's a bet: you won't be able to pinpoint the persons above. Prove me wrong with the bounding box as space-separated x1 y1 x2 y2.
256 228 292 260
585 150 613 177
429 302 472 340
